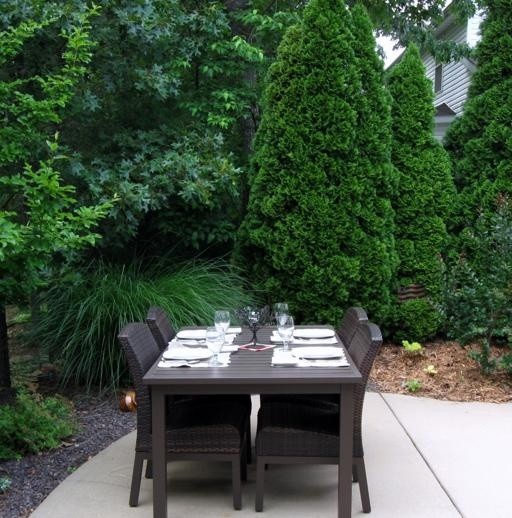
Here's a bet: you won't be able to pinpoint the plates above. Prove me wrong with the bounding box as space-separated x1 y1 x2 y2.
293 328 335 339
291 347 342 360
175 329 207 340
162 351 214 360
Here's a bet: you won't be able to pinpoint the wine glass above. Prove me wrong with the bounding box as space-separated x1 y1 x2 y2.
273 302 289 338
277 316 295 357
214 310 231 346
205 326 226 366
